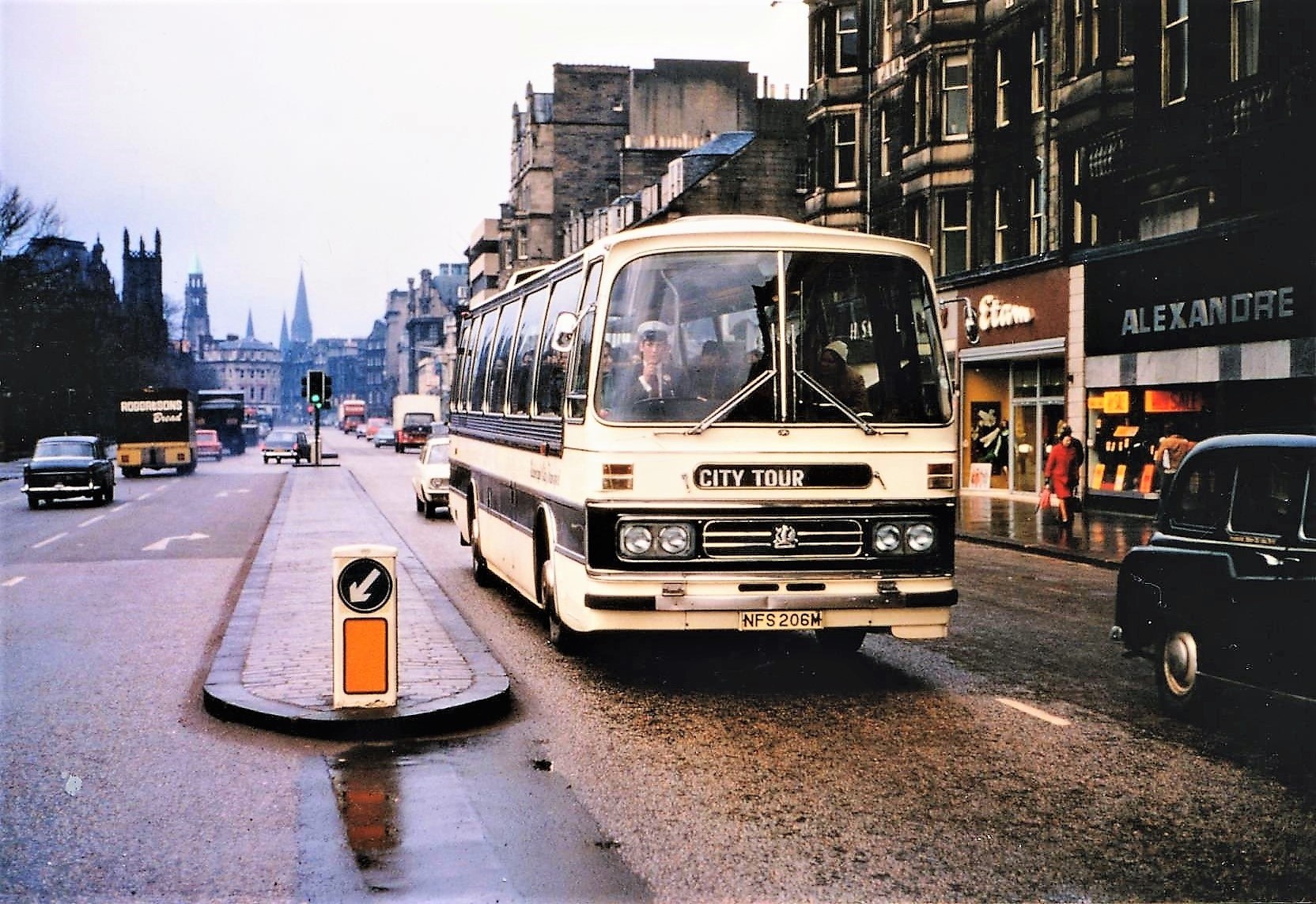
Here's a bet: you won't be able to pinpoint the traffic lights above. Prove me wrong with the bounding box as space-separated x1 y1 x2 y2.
308 370 325 404
324 375 332 408
301 375 309 399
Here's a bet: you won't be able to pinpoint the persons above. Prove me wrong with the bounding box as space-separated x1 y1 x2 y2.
1152 423 1189 522
993 420 1010 479
1058 424 1084 521
490 320 866 419
1044 432 1080 529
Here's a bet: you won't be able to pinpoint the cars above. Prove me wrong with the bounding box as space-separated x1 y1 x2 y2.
20 436 117 510
1105 433 1316 703
194 428 224 461
374 427 396 447
260 430 313 466
367 418 388 442
357 424 369 438
412 436 453 520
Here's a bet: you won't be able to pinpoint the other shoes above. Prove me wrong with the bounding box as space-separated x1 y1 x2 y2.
1057 515 1074 525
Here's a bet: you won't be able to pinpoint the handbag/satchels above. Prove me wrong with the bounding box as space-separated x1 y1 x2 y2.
1161 448 1171 469
1040 489 1052 511
1066 497 1083 514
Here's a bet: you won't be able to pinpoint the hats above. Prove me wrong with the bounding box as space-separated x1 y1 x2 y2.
822 339 852 361
636 320 674 344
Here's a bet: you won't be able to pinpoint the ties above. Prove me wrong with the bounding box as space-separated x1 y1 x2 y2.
651 375 660 397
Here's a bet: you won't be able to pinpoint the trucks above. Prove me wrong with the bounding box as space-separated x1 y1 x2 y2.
337 400 369 435
391 395 445 453
117 388 211 479
243 406 275 447
196 389 251 458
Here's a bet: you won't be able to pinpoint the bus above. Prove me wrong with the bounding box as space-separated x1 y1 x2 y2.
446 214 963 650
